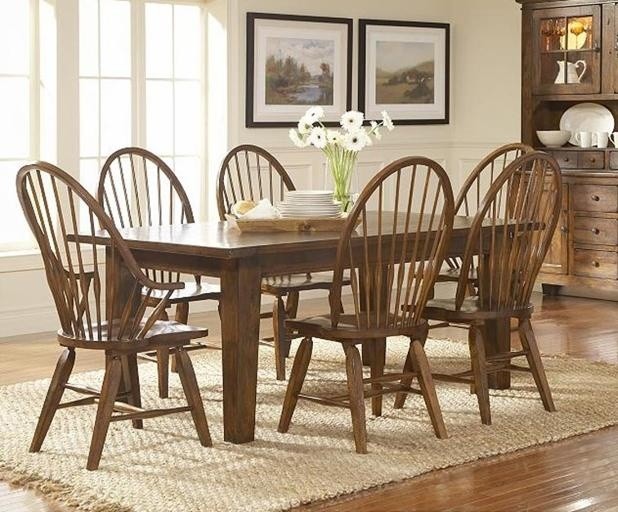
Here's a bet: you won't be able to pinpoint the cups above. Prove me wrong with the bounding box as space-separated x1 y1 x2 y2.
592 132 608 148
608 132 618 148
575 131 593 148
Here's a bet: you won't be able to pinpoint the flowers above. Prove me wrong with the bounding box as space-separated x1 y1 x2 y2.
288 104 394 213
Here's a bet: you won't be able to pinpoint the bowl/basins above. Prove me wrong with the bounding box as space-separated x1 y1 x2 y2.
535 130 572 148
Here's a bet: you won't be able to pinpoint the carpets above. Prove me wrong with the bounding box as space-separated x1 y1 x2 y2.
0 333 618 511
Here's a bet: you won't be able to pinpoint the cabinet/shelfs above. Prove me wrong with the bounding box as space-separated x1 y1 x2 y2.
519 0 603 96
572 184 617 282
603 1 618 93
511 177 569 274
536 148 608 173
608 149 618 170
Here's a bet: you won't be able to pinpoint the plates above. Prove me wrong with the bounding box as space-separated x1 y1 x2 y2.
275 190 346 220
559 102 615 148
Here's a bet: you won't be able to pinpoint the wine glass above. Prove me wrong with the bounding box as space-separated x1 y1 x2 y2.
539 15 593 51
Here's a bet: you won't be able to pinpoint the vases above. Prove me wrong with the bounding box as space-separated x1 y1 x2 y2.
336 196 350 216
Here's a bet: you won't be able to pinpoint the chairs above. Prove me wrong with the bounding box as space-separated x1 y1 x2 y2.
216 144 352 346
394 151 563 425
277 157 454 454
16 161 213 473
97 145 221 398
415 140 542 332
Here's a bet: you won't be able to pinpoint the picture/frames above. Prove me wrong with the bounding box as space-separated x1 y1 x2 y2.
358 18 450 125
245 11 356 130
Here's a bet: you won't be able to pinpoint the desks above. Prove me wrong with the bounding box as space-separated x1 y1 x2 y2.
66 212 546 446
